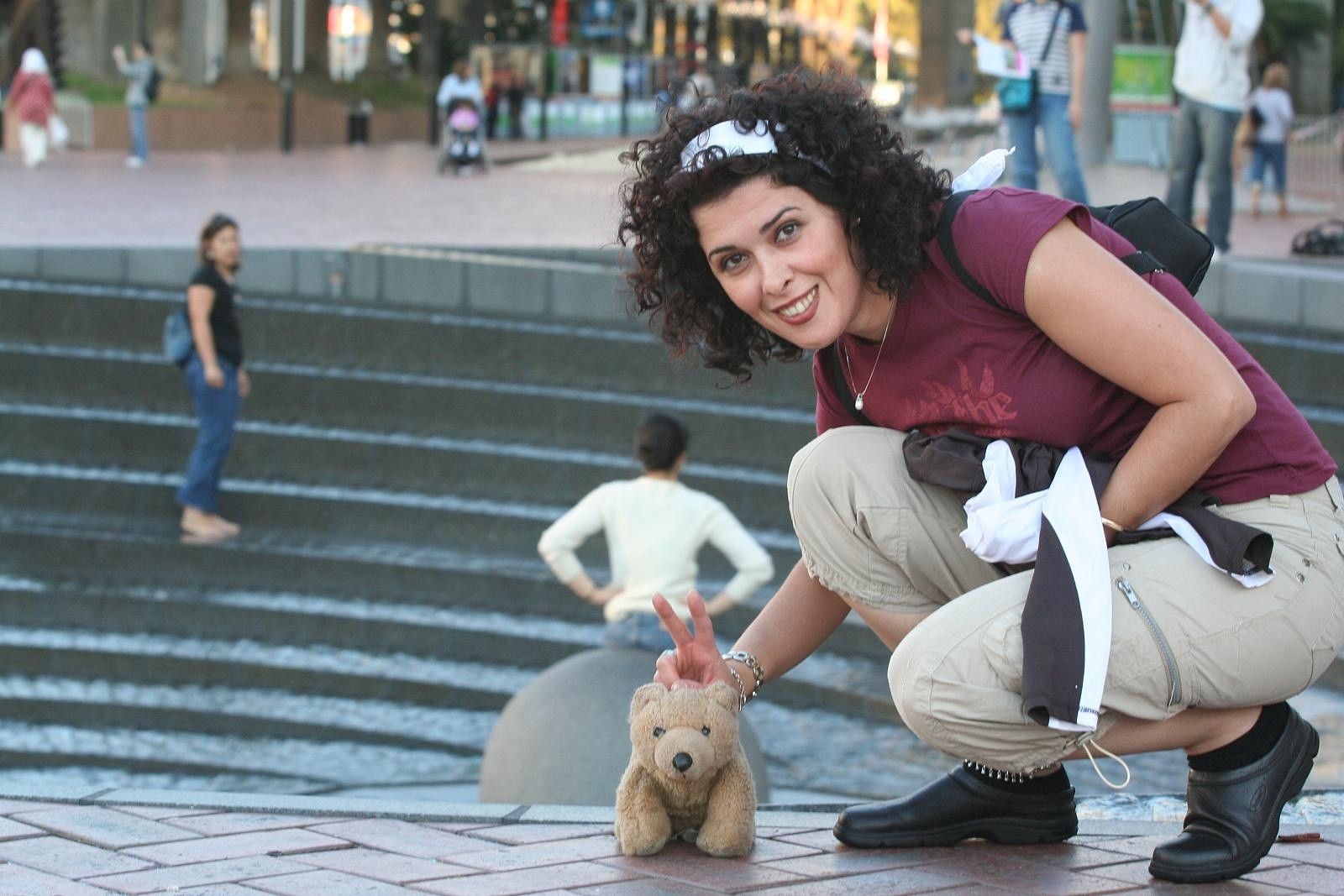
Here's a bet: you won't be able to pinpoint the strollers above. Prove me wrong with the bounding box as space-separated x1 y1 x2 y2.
442 97 490 176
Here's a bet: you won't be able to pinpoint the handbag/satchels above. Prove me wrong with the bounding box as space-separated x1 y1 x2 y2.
997 78 1038 111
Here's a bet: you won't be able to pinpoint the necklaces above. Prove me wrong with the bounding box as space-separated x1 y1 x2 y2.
843 292 897 410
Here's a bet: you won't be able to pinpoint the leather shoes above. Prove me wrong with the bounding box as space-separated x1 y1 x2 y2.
834 767 1078 845
1149 708 1319 876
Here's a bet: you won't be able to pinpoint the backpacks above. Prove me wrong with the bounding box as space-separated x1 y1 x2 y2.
163 312 191 366
1081 196 1216 297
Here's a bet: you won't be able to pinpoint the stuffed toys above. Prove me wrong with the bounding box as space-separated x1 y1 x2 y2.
615 684 756 857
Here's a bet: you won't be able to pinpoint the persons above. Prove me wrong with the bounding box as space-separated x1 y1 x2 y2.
537 415 774 653
1165 0 1263 263
958 0 1089 204
439 59 524 174
10 49 55 168
116 40 154 167
1246 64 1293 219
180 215 250 537
619 73 1344 886
679 66 716 113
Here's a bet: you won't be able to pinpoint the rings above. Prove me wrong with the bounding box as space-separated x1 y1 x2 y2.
661 649 676 658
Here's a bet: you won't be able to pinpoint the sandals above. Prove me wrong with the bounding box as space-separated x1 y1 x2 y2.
179 529 222 543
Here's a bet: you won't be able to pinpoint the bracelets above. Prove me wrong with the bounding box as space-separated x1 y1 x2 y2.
1102 518 1130 532
1204 2 1213 12
722 652 764 711
